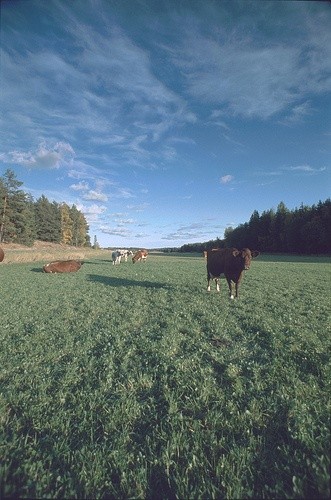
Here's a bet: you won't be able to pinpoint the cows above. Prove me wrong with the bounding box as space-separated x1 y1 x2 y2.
207 248 259 300
41 260 81 273
132 249 148 264
116 250 133 262
112 250 124 266
